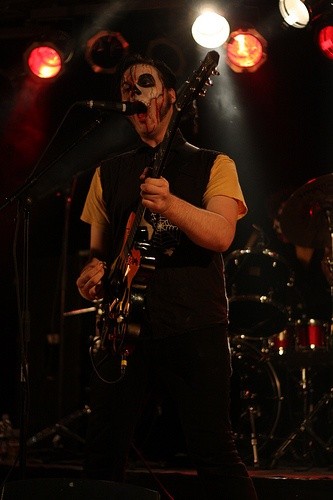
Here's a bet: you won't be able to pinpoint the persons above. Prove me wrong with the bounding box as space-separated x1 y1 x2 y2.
77 53 258 500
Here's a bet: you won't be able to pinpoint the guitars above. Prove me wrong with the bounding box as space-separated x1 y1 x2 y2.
89 50 221 362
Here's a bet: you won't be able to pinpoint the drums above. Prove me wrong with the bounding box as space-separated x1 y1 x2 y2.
222 247 305 335
228 336 304 471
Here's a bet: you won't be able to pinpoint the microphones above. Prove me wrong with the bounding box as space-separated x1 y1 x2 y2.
82 100 137 114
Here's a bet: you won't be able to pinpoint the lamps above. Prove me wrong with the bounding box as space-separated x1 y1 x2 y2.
23 23 83 83
279 0 333 36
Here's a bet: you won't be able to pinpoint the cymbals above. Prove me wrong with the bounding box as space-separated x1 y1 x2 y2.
272 172 333 248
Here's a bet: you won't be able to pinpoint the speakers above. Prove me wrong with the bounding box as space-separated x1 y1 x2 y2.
0 477 161 500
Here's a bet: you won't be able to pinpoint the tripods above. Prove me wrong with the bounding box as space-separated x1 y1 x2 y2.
25 169 92 454
